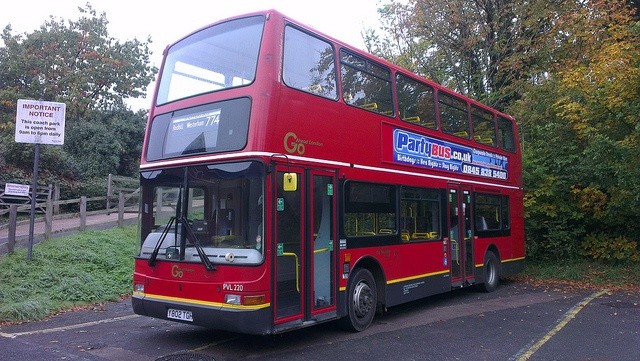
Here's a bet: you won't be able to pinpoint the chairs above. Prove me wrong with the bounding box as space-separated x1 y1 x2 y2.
412 232 428 240
304 83 322 93
453 132 467 138
357 103 378 109
403 235 409 241
430 232 437 239
405 116 420 122
485 138 493 145
475 135 481 141
422 122 437 128
192 221 211 245
383 110 401 115
380 229 391 235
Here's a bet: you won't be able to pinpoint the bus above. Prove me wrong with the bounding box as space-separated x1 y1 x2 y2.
132 8 525 337
